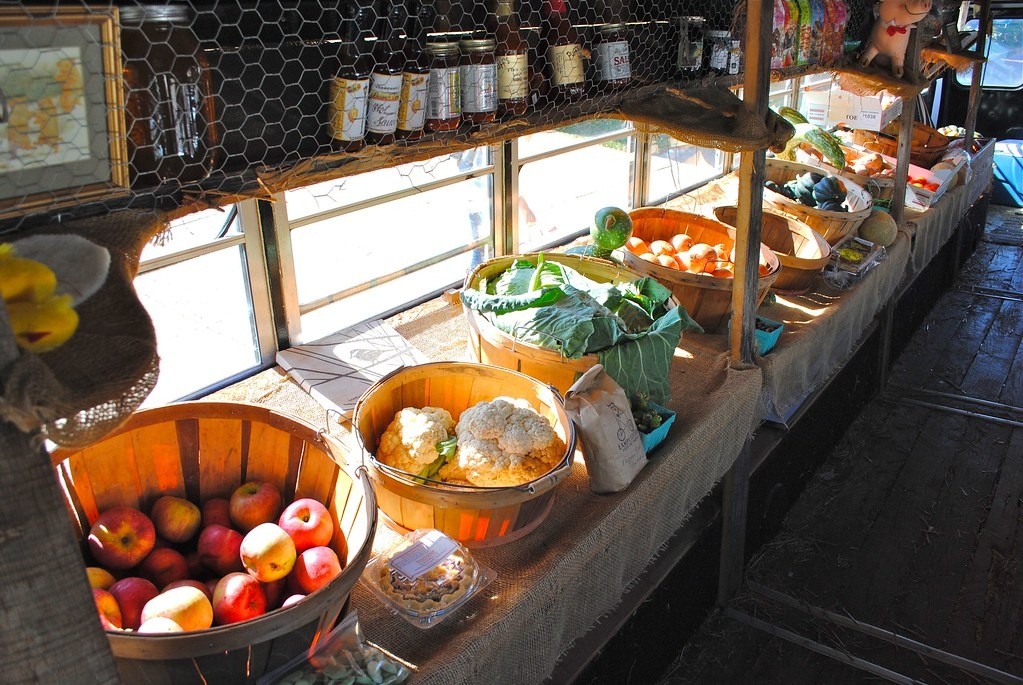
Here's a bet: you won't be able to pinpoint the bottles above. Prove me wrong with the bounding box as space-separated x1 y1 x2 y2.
544 0 586 99
493 0 529 116
707 31 731 72
327 4 370 151
396 4 429 140
364 4 403 145
728 40 741 75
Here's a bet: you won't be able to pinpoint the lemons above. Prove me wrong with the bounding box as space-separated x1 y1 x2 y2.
0 256 80 352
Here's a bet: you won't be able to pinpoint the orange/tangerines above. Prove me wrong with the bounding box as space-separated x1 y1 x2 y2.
627 233 770 278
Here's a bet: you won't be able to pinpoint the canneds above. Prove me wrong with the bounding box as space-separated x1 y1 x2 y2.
426 42 461 132
118 4 215 184
593 22 631 90
670 16 704 80
459 38 498 123
705 30 731 77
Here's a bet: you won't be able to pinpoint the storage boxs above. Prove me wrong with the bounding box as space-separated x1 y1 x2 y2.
803 87 904 133
989 139 1023 209
825 235 884 283
637 401 676 452
728 315 784 355
822 142 967 210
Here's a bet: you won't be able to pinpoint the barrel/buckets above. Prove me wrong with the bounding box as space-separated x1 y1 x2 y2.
41 116 953 684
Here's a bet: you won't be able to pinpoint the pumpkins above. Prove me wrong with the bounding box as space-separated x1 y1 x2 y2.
764 171 846 212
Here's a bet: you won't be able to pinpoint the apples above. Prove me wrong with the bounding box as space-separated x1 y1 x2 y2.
84 481 342 634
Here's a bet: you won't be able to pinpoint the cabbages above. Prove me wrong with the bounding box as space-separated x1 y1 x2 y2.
460 250 704 407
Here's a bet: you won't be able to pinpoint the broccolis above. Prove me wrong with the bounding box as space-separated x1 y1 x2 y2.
377 401 562 491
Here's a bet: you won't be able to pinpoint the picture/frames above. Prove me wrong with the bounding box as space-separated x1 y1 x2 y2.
0 6 131 220
